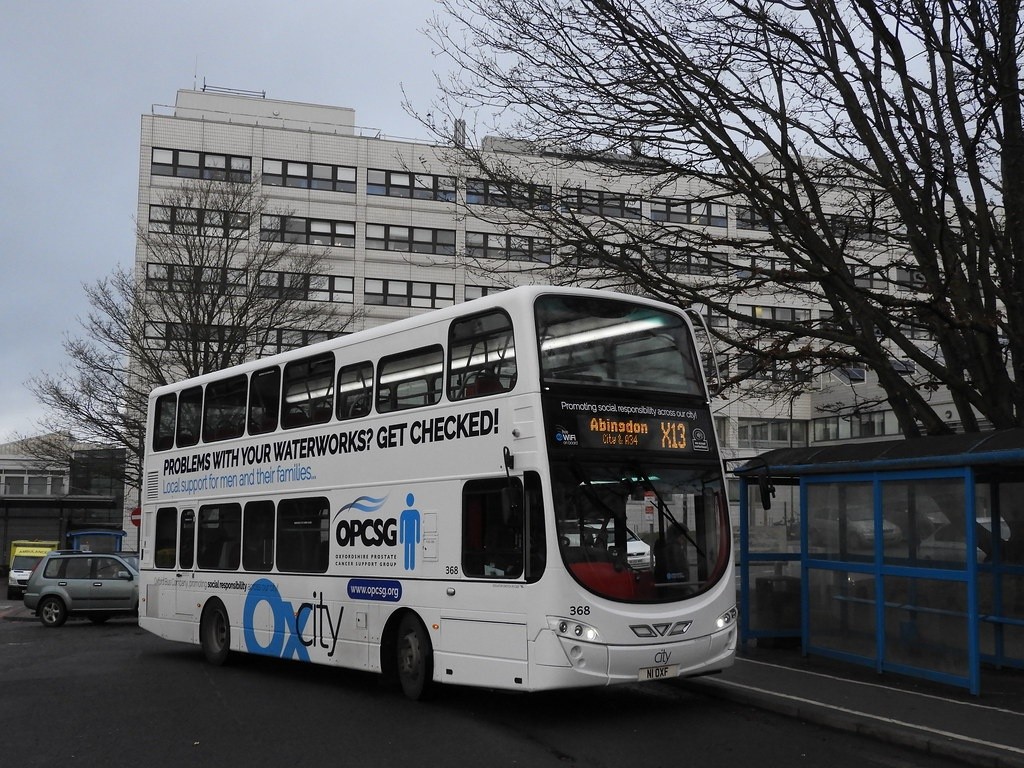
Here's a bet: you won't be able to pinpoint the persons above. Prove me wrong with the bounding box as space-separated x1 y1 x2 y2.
655 523 691 587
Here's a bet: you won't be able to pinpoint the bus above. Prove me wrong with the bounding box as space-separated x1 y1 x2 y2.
137 285 776 704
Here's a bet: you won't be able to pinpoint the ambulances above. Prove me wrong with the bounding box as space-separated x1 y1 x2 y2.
6 539 61 600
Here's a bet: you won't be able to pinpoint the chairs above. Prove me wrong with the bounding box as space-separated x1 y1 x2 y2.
153 369 516 452
218 540 237 570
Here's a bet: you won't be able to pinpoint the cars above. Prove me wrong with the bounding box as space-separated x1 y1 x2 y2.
23 549 139 629
559 521 659 575
917 516 1011 564
808 503 904 551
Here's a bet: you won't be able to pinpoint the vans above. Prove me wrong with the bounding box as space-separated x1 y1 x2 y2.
66 529 128 554
